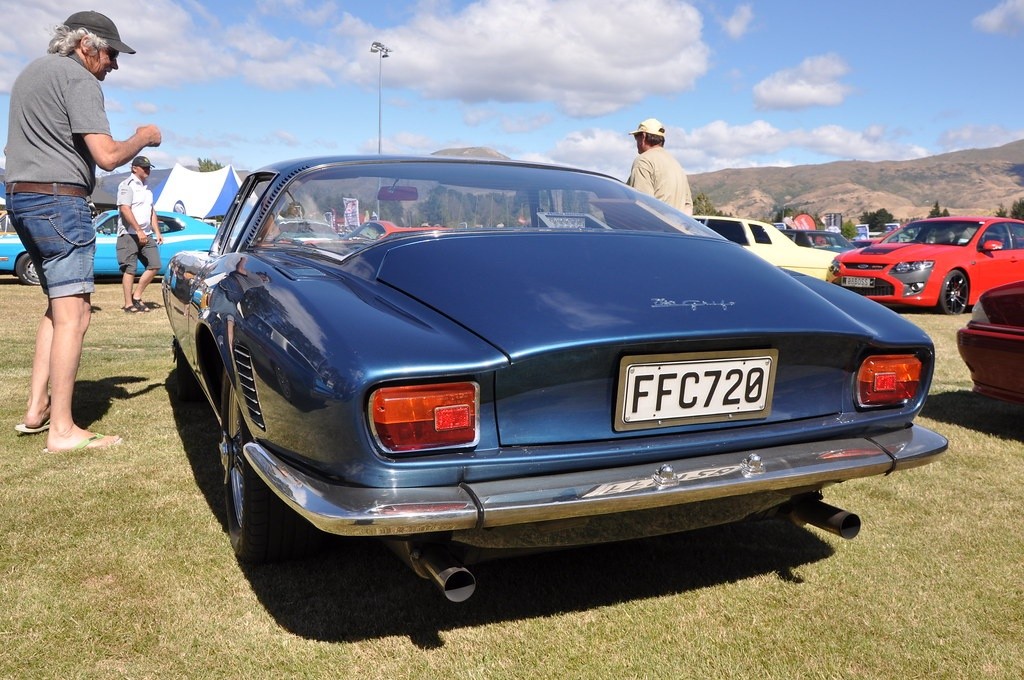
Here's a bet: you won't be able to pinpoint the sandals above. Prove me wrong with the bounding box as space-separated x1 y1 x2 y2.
132 293 151 312
122 305 143 314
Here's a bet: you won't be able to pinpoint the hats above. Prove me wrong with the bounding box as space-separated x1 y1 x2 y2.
131 155 155 169
628 119 666 138
63 10 137 56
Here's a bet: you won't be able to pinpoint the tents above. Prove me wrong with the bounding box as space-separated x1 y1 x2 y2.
148 163 261 219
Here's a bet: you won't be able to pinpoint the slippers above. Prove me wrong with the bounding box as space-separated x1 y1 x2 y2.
15 419 51 435
43 432 123 453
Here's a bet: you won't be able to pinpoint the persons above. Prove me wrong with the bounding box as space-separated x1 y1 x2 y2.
116 156 163 313
625 119 693 222
200 255 272 375
4 10 162 455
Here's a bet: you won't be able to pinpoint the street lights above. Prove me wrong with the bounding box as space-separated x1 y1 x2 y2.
369 41 393 154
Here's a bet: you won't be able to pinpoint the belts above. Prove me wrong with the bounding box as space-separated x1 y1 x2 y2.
5 183 88 200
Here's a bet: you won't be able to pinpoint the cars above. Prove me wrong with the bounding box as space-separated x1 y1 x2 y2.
0 210 220 286
691 215 880 284
275 219 451 244
825 216 1024 315
956 281 1024 406
161 153 951 603
